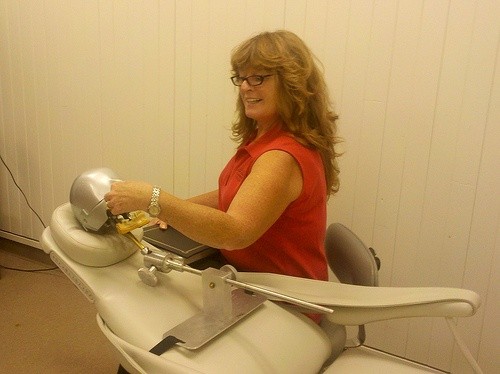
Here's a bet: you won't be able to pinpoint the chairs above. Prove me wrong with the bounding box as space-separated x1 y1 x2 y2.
319 221 382 368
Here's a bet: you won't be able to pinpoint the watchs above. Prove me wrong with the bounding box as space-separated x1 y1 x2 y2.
147 185 160 218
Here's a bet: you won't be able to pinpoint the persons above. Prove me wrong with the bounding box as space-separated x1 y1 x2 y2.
105 28 343 373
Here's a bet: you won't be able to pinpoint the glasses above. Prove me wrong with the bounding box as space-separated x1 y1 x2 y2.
230 73 280 87
